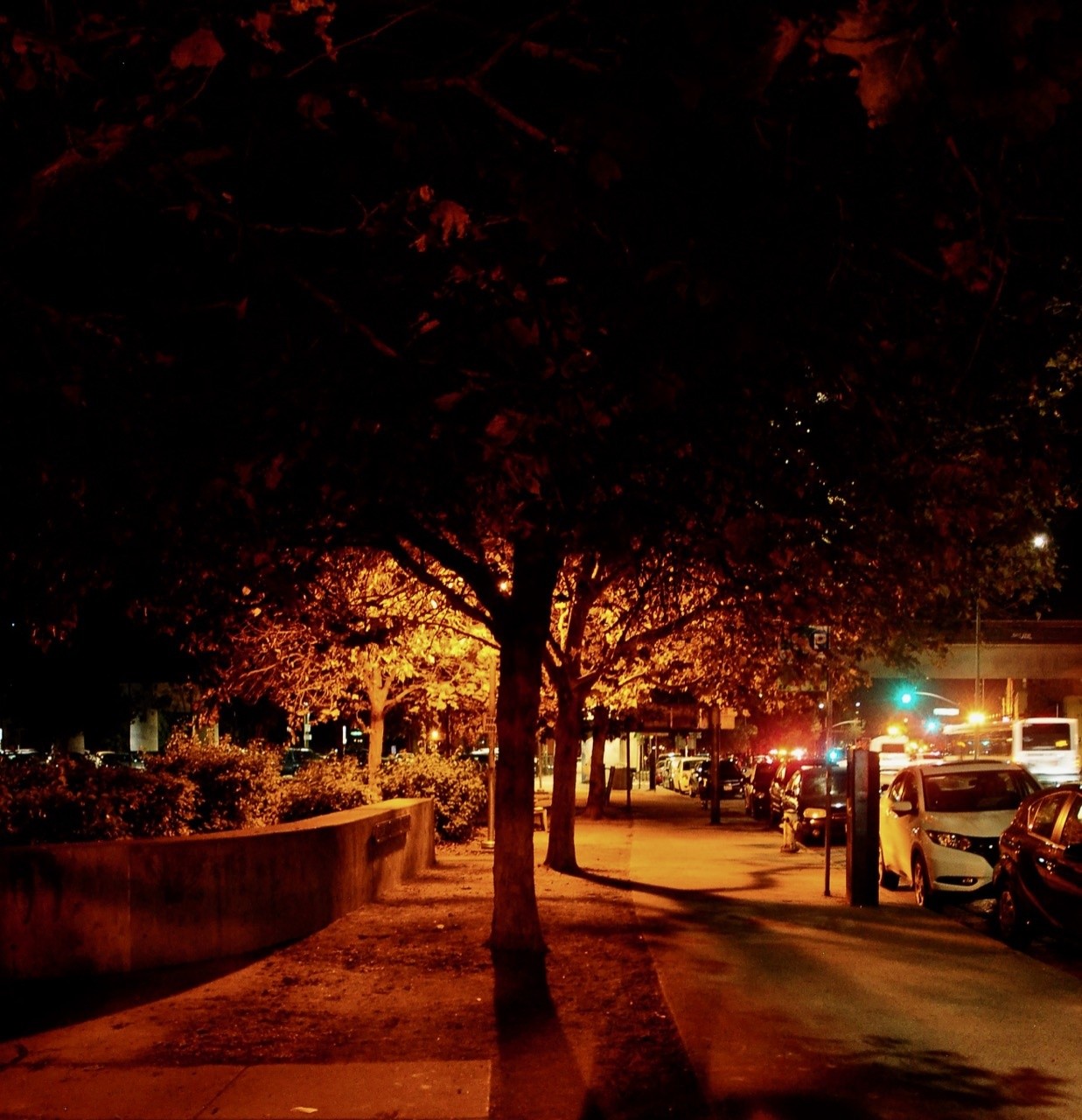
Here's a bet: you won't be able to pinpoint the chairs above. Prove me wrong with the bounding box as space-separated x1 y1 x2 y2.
913 782 945 810
973 775 1012 810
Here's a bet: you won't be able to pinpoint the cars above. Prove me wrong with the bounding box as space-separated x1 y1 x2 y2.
877 759 1045 914
991 779 1082 943
657 732 912 847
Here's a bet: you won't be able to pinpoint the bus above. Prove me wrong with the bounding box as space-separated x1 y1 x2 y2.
940 714 1080 790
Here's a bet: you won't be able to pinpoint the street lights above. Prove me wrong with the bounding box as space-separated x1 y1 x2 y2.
972 531 1048 761
